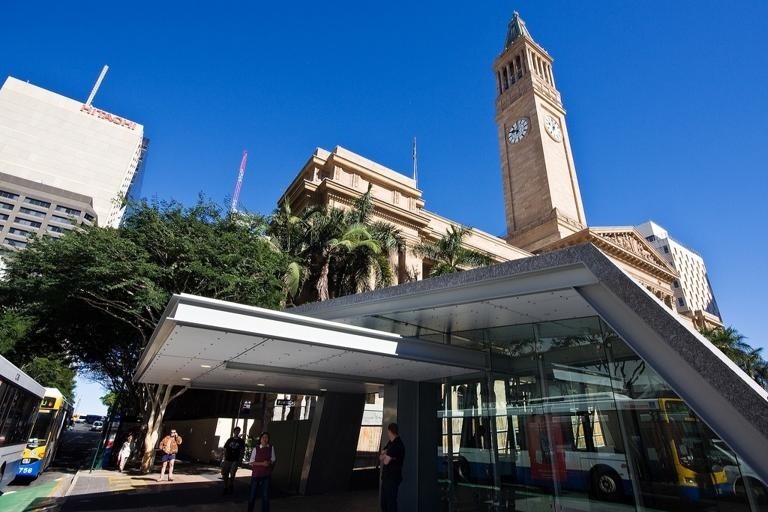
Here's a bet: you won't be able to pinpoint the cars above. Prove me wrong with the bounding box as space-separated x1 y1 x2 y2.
76 415 120 431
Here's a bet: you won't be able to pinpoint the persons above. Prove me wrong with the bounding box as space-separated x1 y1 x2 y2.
157 429 182 482
247 432 276 512
118 431 136 472
379 423 405 512
221 427 245 492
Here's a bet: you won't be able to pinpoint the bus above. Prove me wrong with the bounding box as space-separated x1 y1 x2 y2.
0 355 74 493
